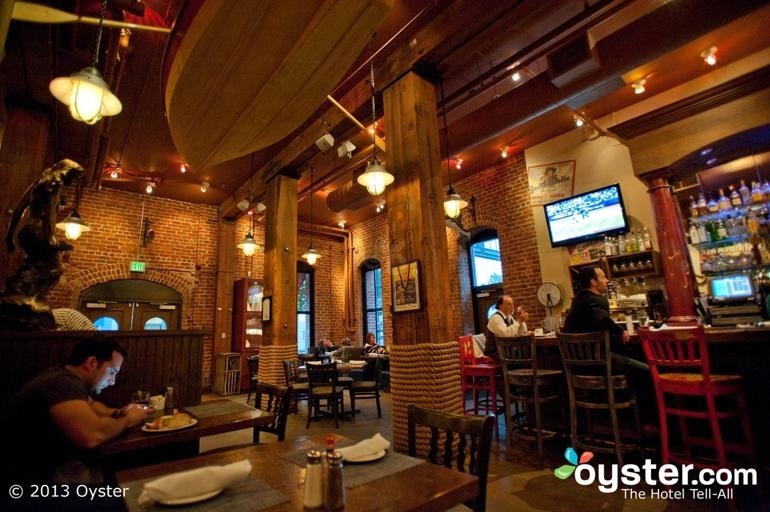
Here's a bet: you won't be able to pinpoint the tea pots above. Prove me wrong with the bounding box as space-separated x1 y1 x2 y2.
540 316 557 332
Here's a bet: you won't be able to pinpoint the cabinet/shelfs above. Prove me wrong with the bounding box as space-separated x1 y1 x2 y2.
670 150 770 326
566 249 664 328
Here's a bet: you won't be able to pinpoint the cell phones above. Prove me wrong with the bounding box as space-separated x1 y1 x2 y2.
143 400 157 410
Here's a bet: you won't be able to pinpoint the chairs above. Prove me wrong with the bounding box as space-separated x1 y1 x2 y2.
555 330 643 470
639 322 757 481
492 333 562 460
61 333 501 512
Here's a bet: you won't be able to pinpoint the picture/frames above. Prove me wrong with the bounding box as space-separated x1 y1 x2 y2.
389 258 427 313
261 295 275 322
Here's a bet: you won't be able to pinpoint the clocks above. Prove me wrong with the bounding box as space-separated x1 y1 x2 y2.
536 281 564 308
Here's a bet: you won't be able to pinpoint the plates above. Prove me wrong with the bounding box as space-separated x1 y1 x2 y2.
141 418 197 431
343 450 386 463
159 484 226 504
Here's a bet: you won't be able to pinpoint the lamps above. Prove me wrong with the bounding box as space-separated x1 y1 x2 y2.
700 46 718 65
236 151 262 259
54 152 92 240
357 59 396 197
574 111 585 124
374 201 385 213
632 78 647 94
301 171 323 269
454 158 463 169
42 14 124 127
336 217 350 228
500 145 511 160
433 60 471 222
105 157 210 199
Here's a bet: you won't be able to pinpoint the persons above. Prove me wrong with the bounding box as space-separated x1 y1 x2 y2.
4 155 86 304
10 333 159 486
562 263 662 443
313 333 387 357
483 294 530 402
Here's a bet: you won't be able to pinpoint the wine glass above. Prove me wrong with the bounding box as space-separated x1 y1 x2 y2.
611 259 654 273
613 275 648 294
698 239 752 272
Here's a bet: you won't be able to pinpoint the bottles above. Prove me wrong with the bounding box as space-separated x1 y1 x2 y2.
638 315 647 328
571 247 591 263
302 435 347 511
164 386 175 415
753 321 770 327
603 224 652 257
686 178 770 245
756 237 770 266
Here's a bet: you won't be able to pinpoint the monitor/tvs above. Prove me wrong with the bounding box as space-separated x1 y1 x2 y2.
543 183 630 248
707 274 756 303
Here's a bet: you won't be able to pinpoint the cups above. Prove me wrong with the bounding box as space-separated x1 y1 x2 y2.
535 328 543 336
132 389 151 404
